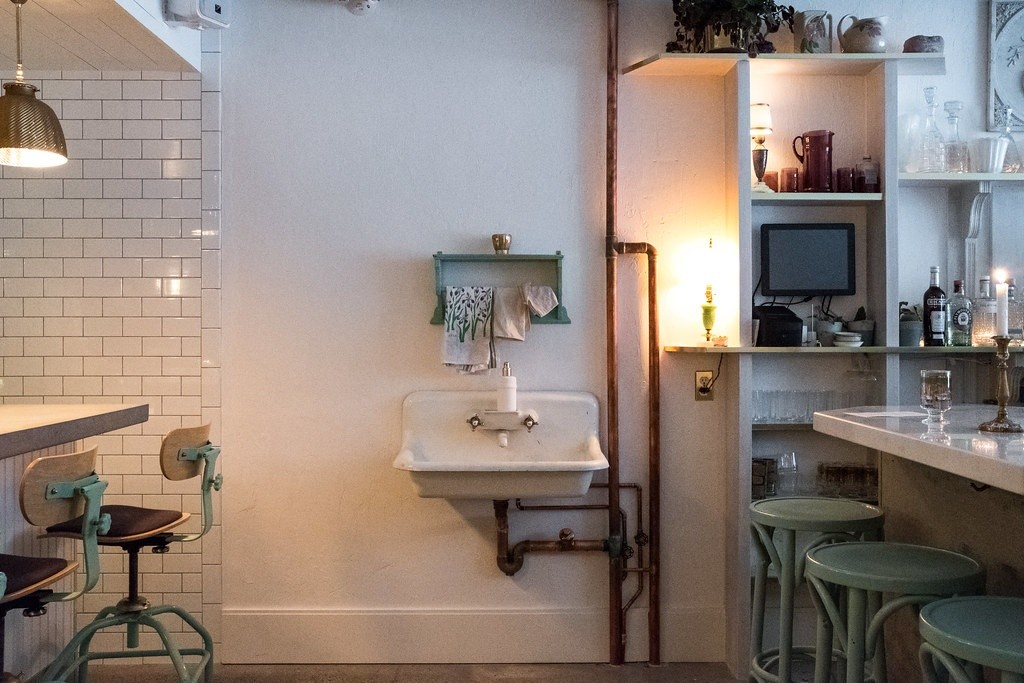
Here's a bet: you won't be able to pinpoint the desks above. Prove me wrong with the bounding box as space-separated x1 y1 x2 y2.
0 404 149 683
810 403 1024 683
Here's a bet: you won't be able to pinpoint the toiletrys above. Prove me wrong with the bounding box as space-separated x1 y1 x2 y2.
497 361 517 412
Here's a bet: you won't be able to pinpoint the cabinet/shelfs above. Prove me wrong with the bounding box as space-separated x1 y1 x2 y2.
429 249 572 326
618 52 1024 683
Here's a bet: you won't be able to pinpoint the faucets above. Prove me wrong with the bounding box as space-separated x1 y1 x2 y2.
497 430 508 448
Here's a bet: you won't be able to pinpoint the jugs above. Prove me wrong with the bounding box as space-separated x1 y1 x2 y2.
836 13 891 53
792 129 835 193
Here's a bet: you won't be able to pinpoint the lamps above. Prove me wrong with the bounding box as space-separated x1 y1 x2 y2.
697 235 719 347
749 102 778 194
0 0 70 169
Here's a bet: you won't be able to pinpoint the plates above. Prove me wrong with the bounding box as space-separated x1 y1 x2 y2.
833 331 864 347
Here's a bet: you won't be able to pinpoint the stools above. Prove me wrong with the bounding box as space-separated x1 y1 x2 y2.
916 595 1024 683
802 540 985 683
745 494 889 683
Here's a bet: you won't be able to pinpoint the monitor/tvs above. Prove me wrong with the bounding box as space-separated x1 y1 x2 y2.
761 222 857 296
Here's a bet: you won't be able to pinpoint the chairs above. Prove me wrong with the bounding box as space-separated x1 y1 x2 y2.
0 443 112 683
34 415 225 683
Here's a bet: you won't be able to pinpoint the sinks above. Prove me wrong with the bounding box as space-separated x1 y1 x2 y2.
392 388 610 501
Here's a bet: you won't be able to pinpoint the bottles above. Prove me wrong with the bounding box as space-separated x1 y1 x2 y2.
998 105 1021 173
898 87 969 174
923 266 947 346
971 275 996 347
946 279 973 346
1008 278 1024 345
844 370 879 408
856 154 881 193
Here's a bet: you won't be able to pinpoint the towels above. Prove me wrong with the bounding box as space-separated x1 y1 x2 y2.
492 287 531 341
517 282 559 318
442 285 498 376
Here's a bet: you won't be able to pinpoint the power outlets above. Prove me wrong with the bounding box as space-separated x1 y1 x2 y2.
695 370 714 401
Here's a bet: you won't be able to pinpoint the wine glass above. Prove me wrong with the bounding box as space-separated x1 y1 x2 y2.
919 370 953 424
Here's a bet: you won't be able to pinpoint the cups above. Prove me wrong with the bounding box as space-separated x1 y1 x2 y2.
762 171 778 193
752 389 837 423
492 234 511 255
837 167 854 193
971 139 1008 171
816 460 877 496
780 168 799 193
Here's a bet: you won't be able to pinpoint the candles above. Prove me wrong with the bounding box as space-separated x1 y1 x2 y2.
991 266 1009 337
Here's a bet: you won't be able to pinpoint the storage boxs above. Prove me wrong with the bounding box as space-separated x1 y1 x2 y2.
752 457 778 500
815 460 878 500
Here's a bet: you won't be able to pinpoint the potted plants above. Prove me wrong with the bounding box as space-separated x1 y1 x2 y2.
664 0 797 59
